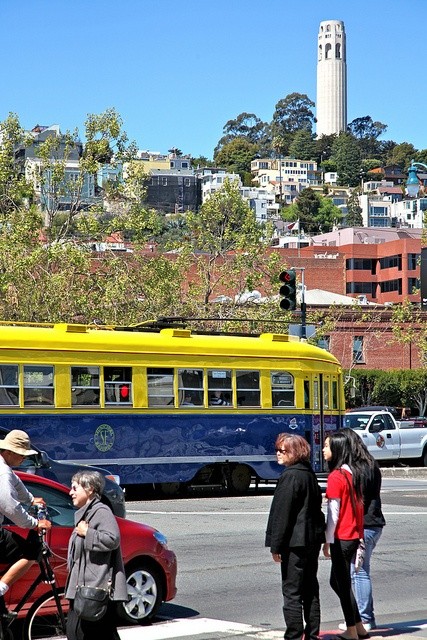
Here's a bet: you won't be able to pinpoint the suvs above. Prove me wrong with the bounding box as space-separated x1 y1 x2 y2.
0 425 126 520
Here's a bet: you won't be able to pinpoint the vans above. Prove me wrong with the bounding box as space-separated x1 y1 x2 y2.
350 406 399 421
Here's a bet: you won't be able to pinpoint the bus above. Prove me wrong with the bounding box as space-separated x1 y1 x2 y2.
0 318 347 502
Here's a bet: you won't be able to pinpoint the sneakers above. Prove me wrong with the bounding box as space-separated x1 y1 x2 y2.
344 620 376 629
0 608 18 619
338 623 371 631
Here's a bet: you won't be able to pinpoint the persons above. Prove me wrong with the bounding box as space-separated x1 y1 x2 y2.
182 394 194 405
264 432 326 639
208 391 240 406
322 433 371 640
0 430 54 618
64 470 129 640
334 427 386 632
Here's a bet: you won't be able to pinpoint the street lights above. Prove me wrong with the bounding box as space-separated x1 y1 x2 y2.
405 160 427 198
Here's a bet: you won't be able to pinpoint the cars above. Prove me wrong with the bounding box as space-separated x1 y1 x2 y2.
0 469 178 625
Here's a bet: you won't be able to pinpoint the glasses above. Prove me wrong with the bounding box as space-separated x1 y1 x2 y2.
72 485 81 492
274 448 285 453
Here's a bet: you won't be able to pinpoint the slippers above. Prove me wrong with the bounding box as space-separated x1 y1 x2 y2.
329 634 356 640
357 633 370 639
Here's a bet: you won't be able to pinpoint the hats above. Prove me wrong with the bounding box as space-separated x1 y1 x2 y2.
0 429 39 456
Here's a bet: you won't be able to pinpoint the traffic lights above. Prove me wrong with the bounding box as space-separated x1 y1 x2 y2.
278 270 295 310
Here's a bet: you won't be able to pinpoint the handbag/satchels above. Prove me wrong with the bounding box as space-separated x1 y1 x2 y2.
354 541 366 574
73 584 118 622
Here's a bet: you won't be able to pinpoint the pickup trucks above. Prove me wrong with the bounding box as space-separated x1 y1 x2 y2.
343 410 427 468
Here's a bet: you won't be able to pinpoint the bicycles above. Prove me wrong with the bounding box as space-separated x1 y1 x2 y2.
0 500 71 640
